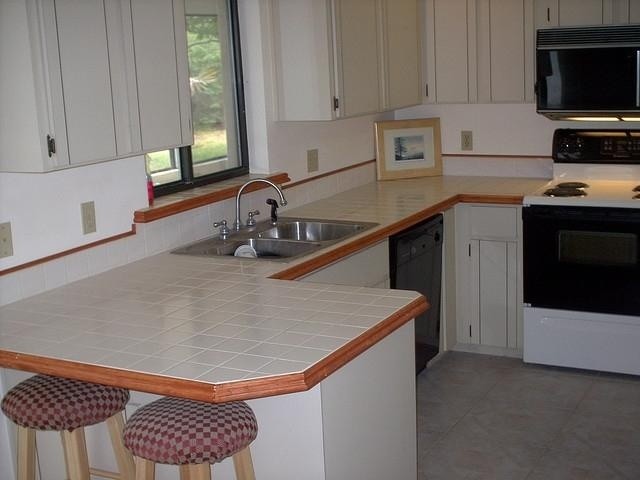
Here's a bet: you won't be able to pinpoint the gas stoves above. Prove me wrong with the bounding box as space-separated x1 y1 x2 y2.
521 175 639 212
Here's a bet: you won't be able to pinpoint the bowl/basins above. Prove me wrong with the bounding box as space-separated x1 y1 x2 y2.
233 244 256 259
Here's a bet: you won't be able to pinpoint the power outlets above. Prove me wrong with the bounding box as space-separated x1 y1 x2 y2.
461 130 473 151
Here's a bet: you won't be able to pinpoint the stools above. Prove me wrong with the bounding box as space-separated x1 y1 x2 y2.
1 373 134 480
124 393 261 480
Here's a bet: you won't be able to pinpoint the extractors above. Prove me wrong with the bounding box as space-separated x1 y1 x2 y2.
533 26 640 124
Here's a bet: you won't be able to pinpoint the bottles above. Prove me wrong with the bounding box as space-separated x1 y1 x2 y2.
146 172 154 207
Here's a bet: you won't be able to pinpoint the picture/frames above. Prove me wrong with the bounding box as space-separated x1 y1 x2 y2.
374 117 443 182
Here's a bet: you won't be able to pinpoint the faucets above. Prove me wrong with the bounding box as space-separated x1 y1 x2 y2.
233 178 288 232
265 198 279 222
168 219 380 264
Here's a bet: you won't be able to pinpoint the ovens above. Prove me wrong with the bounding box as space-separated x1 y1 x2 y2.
522 205 638 316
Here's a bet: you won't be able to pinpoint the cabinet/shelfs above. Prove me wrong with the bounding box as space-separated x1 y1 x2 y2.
455 193 523 361
425 1 532 103
263 0 425 122
533 0 640 29
294 226 391 290
1 0 195 173
